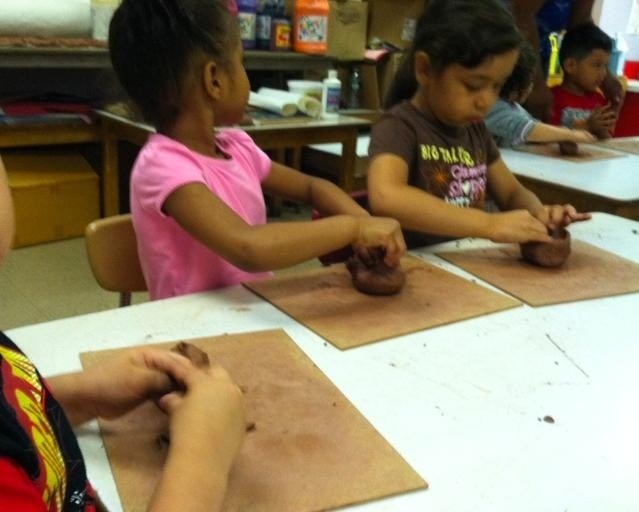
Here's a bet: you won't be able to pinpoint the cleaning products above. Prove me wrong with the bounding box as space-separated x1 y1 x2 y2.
322 70 342 116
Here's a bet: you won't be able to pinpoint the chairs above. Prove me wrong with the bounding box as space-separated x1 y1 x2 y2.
86 214 149 306
314 191 370 266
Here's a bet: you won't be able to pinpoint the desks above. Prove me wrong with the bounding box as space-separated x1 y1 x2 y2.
90 101 373 219
498 133 639 224
0 211 639 512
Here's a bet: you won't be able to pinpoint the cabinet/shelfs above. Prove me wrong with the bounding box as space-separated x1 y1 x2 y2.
0 46 335 222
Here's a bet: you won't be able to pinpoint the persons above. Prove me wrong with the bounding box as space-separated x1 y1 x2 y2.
363 0 594 257
545 20 625 144
106 2 409 308
0 154 256 512
479 42 598 161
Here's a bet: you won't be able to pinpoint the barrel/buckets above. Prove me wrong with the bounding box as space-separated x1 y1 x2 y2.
293 0 329 54
0 0 94 42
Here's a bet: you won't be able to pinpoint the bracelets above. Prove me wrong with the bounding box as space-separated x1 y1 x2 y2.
610 95 621 104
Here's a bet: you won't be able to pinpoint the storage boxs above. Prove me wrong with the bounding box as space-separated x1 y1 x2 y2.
327 0 426 109
0 154 101 249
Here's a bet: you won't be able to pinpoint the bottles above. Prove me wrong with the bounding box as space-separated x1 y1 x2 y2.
320 70 341 119
345 67 364 109
236 0 292 52
